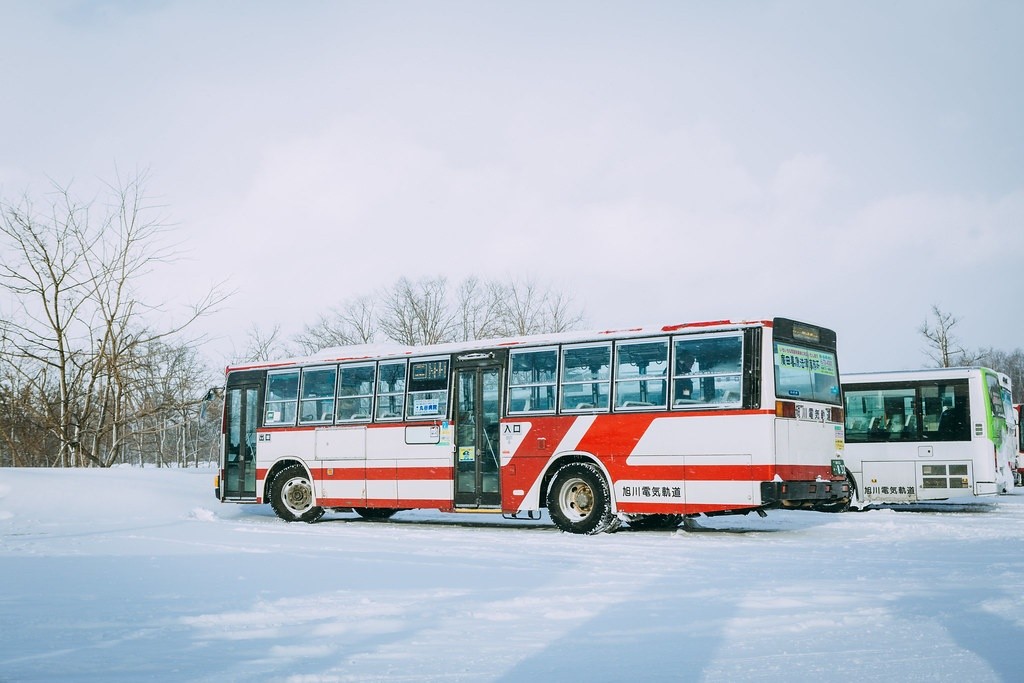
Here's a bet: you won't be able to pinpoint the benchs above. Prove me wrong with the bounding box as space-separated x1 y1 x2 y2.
674 399 706 405
381 412 401 418
624 401 655 407
352 414 367 420
294 415 315 422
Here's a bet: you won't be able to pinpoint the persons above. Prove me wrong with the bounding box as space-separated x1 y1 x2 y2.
662 351 695 405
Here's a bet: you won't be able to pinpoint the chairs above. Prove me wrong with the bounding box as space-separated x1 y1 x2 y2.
868 417 881 431
721 391 740 403
576 403 595 409
321 412 334 421
849 419 863 431
923 415 937 431
883 419 892 431
903 414 917 432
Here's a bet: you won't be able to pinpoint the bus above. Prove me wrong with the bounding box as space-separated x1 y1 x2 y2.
1008 403 1024 489
480 363 1018 511
203 317 849 537
